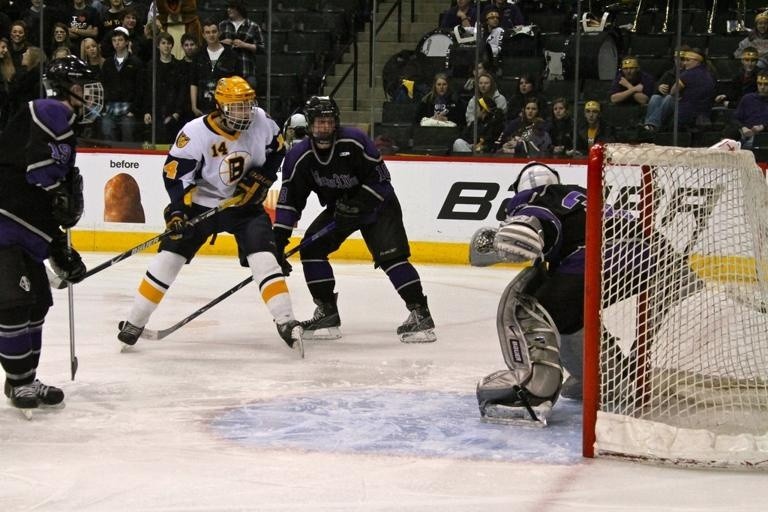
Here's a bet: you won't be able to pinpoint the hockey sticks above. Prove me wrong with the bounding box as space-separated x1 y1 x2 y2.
67 228 78 381
45 192 246 289
118 217 336 340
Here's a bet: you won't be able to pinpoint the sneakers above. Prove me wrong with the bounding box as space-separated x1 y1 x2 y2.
116 319 147 346
396 295 442 336
4 380 38 408
33 379 65 405
273 312 303 351
298 291 342 330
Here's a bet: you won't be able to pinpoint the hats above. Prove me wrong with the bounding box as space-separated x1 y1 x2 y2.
620 57 641 70
111 26 130 37
754 12 768 23
584 99 600 112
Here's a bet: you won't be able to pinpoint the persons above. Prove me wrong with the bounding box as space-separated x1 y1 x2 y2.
283 112 309 157
1 54 95 408
467 163 675 406
272 93 435 335
116 74 304 350
0 0 266 147
415 1 767 162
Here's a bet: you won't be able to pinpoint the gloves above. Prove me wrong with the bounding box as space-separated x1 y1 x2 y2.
46 234 88 286
231 166 279 210
165 209 186 241
268 228 294 278
52 166 87 231
332 183 387 228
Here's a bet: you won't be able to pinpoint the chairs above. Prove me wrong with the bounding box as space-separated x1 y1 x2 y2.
0 1 767 165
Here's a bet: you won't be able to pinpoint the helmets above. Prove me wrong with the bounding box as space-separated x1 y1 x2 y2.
507 161 562 193
302 95 341 130
213 74 257 112
41 54 102 100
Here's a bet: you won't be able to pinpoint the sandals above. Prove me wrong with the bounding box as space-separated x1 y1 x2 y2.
637 122 659 136
